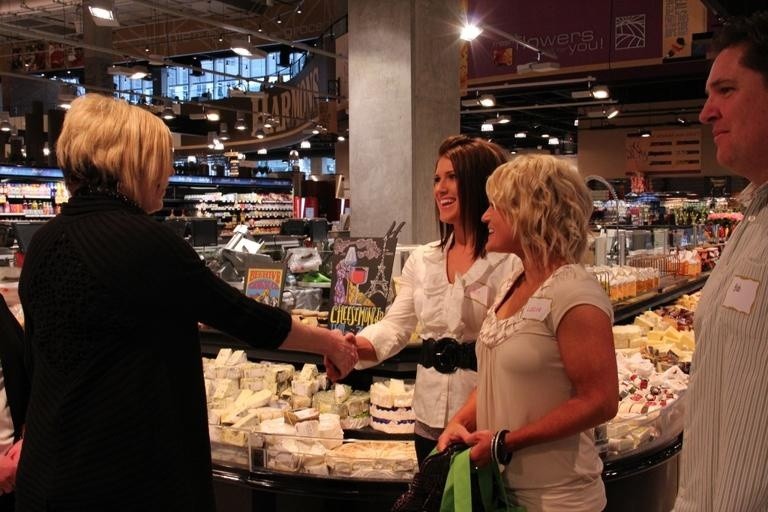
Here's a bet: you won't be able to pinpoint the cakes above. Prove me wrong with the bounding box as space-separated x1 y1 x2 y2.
595 290 700 459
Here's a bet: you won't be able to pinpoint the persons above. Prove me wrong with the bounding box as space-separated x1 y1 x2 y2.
437 152 620 511
666 9 768 512
0 291 31 511
18 94 360 512
322 135 512 473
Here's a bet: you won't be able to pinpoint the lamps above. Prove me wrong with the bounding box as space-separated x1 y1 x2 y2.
0 0 689 164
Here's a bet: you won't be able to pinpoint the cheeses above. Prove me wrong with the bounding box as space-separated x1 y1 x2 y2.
203 349 419 478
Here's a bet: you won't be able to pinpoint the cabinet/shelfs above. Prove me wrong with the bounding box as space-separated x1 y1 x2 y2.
0 171 743 512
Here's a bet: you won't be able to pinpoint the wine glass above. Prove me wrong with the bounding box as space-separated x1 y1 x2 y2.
348 266 370 306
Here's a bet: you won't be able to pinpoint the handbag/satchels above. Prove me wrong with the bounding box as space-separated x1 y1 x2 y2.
391 443 470 508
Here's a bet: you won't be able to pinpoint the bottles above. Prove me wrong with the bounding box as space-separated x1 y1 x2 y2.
679 249 702 276
0 182 65 216
582 262 661 302
626 206 633 224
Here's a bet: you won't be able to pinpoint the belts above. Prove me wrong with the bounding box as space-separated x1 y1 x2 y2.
420 338 475 374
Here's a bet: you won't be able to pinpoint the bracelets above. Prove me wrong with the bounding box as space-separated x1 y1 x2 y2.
490 427 513 473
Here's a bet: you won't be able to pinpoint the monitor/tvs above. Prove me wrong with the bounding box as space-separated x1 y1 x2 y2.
310 217 328 243
191 218 220 246
